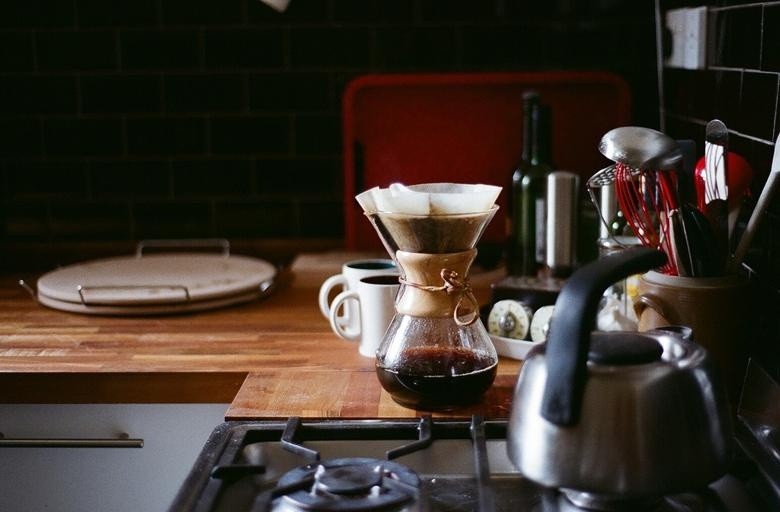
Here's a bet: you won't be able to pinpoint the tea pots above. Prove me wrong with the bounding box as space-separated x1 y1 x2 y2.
507 247 736 499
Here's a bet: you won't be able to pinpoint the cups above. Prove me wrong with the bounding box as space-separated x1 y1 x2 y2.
318 259 403 360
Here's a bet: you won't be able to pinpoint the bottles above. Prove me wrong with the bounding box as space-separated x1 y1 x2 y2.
364 210 500 410
507 89 581 278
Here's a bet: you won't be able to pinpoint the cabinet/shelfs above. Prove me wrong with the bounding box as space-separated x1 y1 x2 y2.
0 402 230 512
665 0 779 146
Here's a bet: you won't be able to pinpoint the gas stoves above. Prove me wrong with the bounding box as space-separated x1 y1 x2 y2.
165 416 751 511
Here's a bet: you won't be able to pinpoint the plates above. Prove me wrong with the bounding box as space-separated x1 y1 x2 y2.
488 335 543 362
